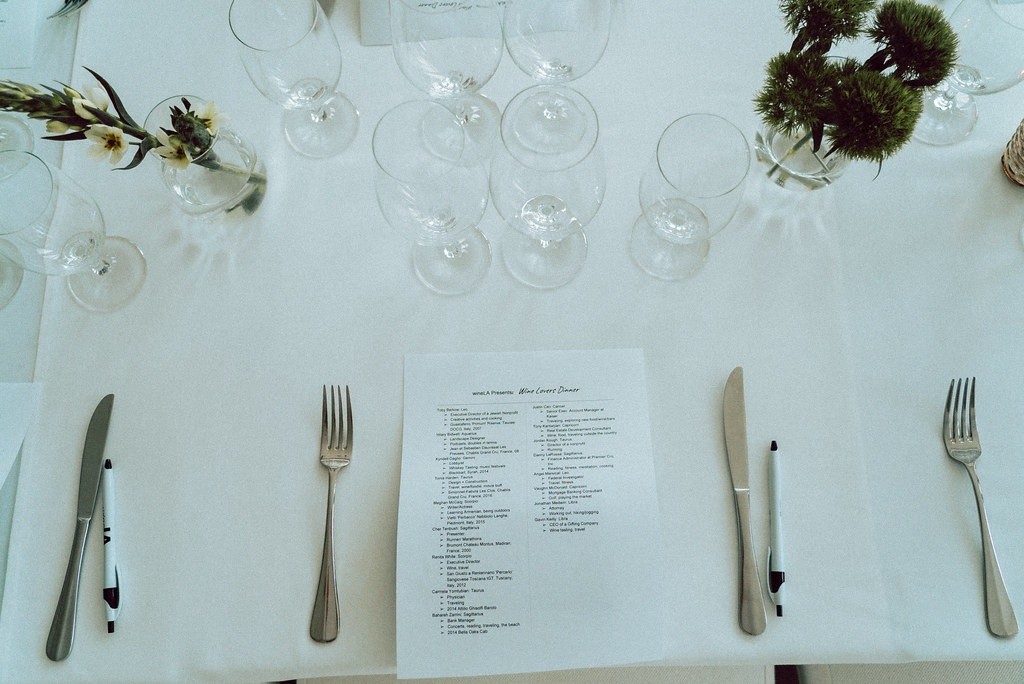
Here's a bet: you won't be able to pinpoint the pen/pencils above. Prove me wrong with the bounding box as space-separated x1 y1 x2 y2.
101 458 120 634
766 441 787 617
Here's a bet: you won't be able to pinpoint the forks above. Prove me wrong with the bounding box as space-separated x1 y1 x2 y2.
308 384 354 644
942 376 1022 638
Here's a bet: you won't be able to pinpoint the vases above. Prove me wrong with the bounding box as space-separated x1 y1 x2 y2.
754 55 864 191
142 94 269 215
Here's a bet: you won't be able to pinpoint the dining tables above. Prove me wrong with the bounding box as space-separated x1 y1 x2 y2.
0 1 1024 684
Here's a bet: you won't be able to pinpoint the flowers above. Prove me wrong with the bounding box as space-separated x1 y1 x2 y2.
0 64 268 214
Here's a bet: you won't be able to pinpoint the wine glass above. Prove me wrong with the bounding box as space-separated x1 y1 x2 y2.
1 1 1024 317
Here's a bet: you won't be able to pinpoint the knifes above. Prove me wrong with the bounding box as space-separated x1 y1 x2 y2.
45 392 114 662
720 364 767 636
